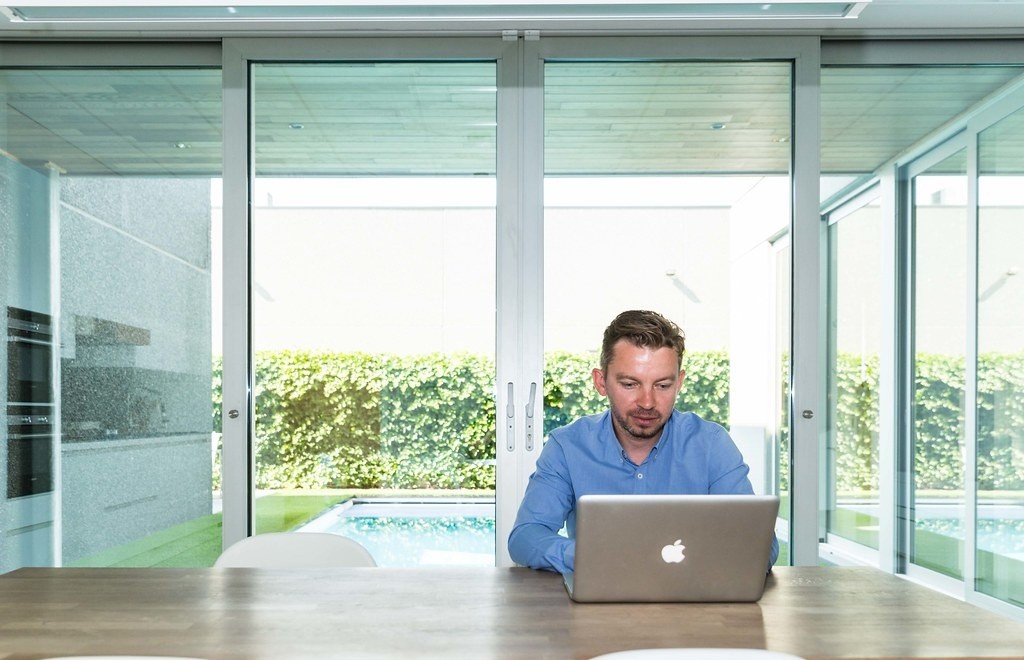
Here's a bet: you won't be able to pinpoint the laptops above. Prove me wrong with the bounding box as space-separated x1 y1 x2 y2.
563 495 780 603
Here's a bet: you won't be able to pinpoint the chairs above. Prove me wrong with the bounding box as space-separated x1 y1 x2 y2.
213 531 377 567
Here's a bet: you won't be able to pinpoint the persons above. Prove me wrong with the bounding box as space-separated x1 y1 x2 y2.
506 311 780 574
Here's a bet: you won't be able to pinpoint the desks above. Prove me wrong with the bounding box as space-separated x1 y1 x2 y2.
0 566 1024 660
